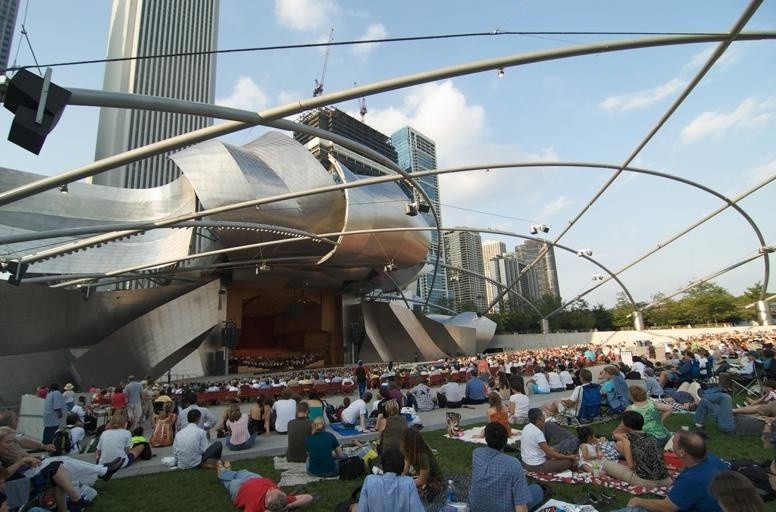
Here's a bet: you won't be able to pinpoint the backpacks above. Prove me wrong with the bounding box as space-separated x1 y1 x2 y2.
48 424 82 456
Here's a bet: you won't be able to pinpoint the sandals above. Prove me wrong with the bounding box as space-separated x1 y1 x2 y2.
680 425 704 432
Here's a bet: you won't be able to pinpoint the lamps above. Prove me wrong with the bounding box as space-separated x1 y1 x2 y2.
404 185 431 217
0 65 73 158
56 183 68 194
384 258 398 272
254 259 271 276
528 222 549 234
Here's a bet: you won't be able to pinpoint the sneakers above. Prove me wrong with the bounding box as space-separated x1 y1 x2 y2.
217 460 223 479
572 489 616 509
697 389 705 398
224 460 231 469
68 495 90 505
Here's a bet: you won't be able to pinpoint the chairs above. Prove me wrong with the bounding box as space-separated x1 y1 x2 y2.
168 357 590 404
654 352 775 400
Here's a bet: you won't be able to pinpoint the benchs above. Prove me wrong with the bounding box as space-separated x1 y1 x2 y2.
0 449 55 512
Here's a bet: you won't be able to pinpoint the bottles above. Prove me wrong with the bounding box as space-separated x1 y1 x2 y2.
445 479 458 505
203 415 210 433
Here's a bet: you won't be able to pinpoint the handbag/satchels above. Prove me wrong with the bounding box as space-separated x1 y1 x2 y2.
446 412 461 437
326 405 335 423
626 371 641 379
133 442 151 460
339 456 364 481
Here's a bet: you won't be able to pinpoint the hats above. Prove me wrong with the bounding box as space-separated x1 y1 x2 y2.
744 350 758 359
64 383 74 390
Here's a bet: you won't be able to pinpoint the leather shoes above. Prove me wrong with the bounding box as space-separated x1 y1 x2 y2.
98 457 124 482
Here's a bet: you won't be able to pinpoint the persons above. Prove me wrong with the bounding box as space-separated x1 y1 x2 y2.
0 321 775 512
